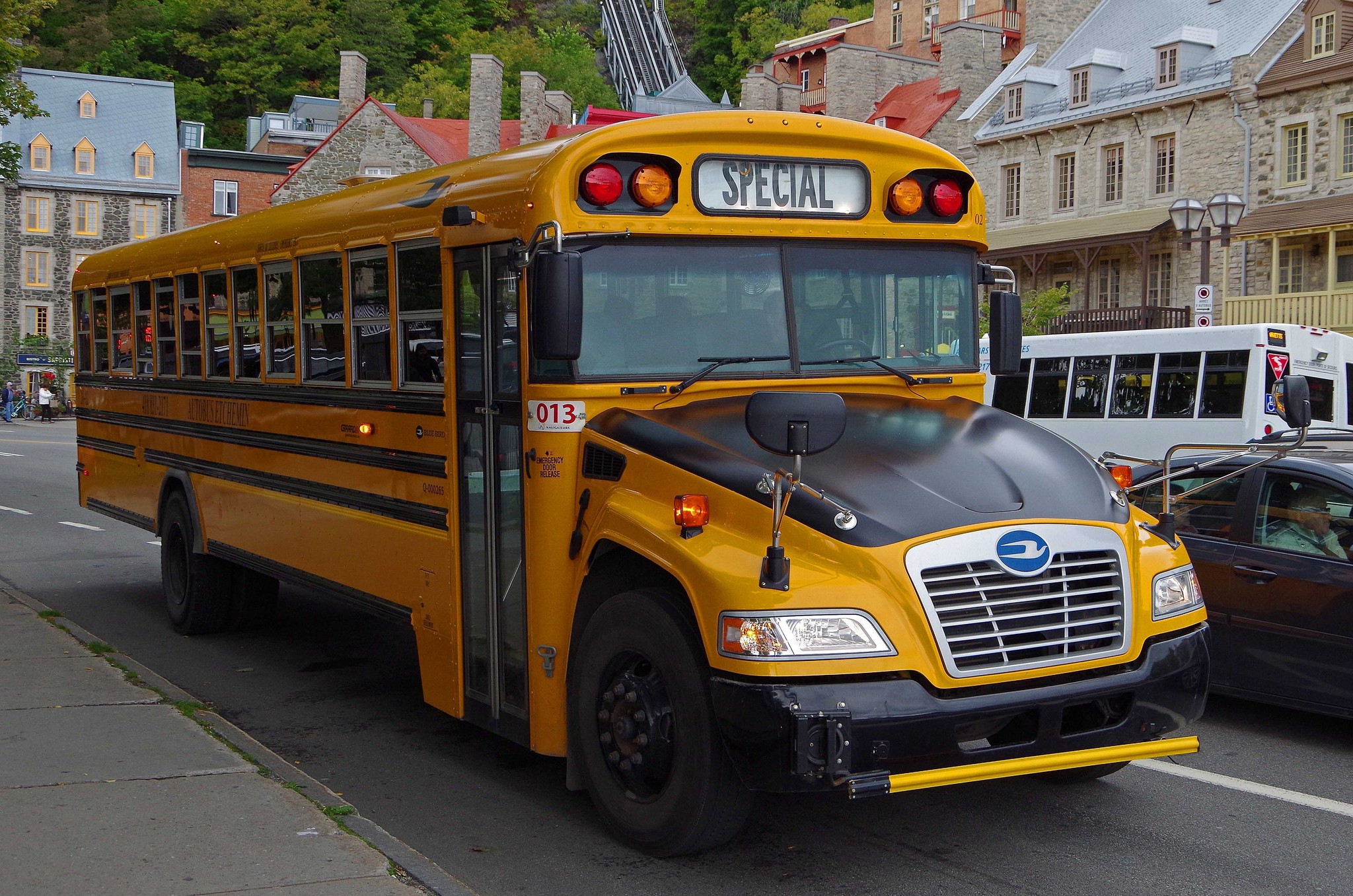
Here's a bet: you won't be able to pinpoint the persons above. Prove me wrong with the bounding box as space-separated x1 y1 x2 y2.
39 383 55 424
2 382 26 423
1151 484 1348 559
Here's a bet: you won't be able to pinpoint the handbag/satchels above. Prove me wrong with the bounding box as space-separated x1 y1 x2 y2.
50 397 59 408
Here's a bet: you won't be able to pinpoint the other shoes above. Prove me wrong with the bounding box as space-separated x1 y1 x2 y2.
41 421 46 424
48 421 55 423
3 417 7 422
7 421 13 423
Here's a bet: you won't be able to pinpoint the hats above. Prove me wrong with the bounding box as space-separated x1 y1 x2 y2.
7 382 13 387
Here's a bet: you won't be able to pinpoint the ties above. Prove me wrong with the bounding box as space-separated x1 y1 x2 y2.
1317 536 1326 547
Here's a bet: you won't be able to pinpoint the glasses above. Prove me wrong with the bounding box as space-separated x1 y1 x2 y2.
1305 508 1331 513
1170 502 1187 510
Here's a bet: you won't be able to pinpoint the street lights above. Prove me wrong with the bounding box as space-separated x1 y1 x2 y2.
1168 193 1246 327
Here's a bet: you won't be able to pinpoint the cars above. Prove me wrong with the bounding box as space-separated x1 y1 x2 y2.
1111 426 1353 720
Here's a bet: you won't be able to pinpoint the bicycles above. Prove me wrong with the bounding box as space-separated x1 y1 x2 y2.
0 396 37 421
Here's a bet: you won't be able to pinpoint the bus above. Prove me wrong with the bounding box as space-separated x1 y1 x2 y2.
75 110 1212 862
951 323 1353 470
